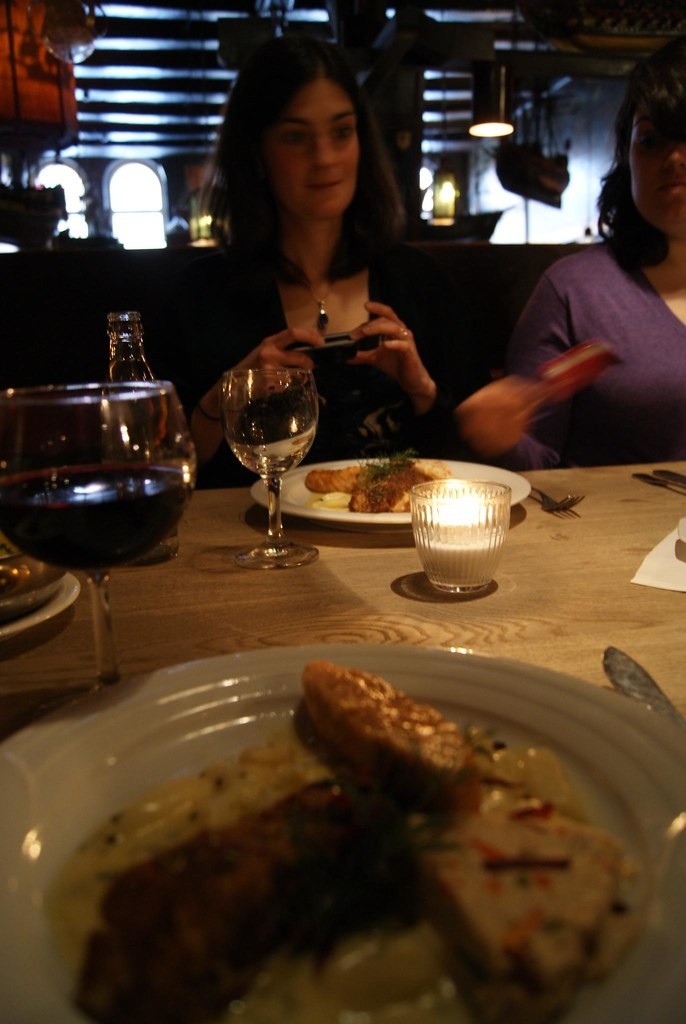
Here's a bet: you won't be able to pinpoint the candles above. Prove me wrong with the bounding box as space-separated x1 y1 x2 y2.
409 478 512 596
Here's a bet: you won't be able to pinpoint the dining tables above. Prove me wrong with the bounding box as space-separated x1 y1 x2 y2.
0 461 686 744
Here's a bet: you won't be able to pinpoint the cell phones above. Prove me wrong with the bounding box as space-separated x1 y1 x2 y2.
292 339 358 364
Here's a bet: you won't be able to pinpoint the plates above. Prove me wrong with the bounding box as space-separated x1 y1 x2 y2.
0 574 81 638
250 459 531 535
0 638 685 1023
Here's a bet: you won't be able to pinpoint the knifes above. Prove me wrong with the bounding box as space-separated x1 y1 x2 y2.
601 645 685 752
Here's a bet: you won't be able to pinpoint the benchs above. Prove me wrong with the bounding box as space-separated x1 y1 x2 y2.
0 244 642 467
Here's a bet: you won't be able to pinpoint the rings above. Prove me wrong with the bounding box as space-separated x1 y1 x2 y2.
400 328 408 341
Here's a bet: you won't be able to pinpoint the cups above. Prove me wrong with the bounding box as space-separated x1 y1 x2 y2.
410 478 514 593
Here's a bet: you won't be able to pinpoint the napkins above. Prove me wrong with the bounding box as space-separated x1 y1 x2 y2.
630 527 686 592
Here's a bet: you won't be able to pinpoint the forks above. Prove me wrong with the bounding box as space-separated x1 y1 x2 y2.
530 484 585 512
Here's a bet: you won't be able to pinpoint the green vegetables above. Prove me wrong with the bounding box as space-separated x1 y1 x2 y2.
355 448 419 499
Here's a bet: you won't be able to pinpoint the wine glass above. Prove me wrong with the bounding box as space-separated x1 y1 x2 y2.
222 367 319 571
0 375 195 716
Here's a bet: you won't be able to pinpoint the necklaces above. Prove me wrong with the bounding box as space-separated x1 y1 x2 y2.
307 284 335 329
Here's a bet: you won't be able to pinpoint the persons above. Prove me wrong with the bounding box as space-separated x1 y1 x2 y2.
457 38 686 471
147 28 475 482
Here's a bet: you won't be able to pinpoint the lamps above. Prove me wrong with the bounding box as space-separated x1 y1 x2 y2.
432 168 468 219
468 64 518 139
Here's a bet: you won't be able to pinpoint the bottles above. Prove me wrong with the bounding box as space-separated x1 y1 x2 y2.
101 310 180 567
